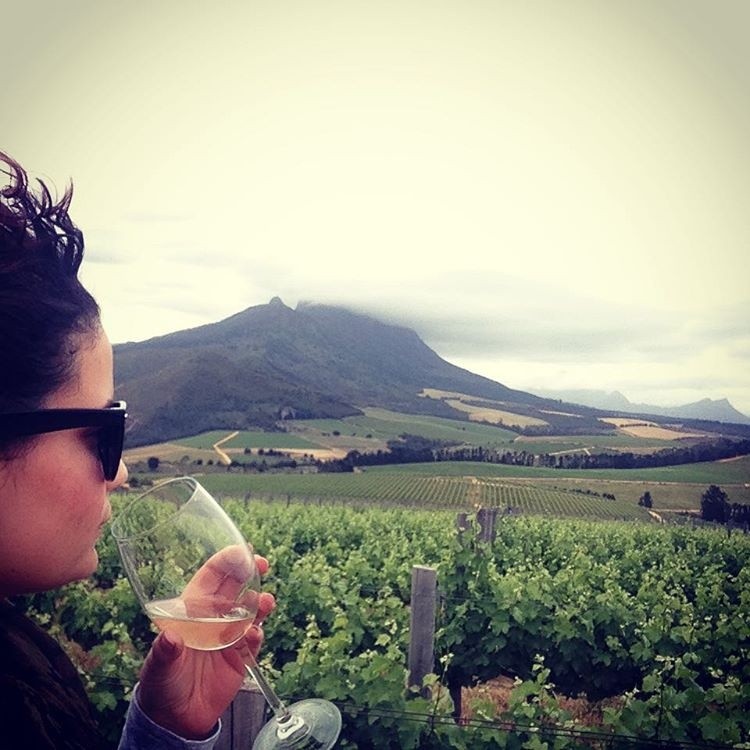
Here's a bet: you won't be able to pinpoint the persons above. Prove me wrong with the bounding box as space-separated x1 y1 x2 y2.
0 151 275 750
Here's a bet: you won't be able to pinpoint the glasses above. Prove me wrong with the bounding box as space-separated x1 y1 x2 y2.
0 400 128 482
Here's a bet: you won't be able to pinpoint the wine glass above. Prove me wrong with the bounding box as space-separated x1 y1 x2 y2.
110 476 342 750
